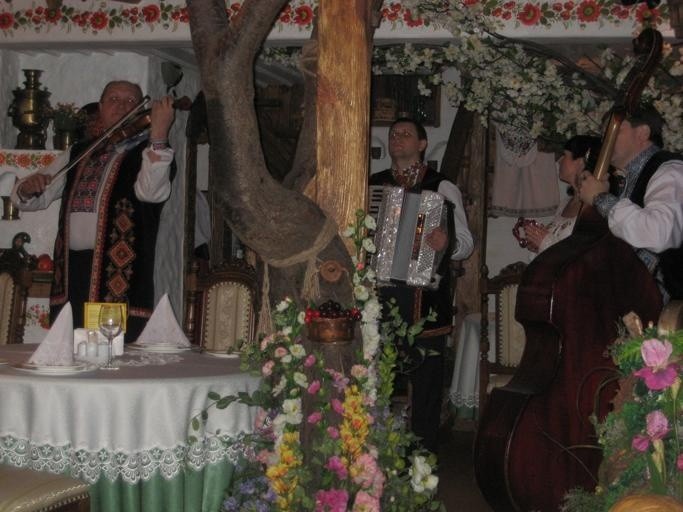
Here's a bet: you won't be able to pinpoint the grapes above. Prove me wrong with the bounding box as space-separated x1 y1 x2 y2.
304 300 362 324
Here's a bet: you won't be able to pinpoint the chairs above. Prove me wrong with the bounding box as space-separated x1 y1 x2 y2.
0 265 26 344
0 463 91 512
478 262 529 417
197 257 262 354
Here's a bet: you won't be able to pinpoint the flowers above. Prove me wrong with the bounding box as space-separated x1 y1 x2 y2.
41 102 88 130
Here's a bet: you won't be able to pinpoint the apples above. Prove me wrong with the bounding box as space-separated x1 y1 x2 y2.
37 254 53 271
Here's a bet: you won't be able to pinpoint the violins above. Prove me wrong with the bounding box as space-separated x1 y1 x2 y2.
84 95 193 153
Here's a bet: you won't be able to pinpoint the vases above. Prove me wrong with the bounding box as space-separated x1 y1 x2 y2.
53 131 79 150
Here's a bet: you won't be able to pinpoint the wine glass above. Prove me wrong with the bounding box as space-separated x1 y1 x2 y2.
98 304 122 372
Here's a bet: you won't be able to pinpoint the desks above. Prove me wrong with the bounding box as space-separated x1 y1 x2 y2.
0 344 266 512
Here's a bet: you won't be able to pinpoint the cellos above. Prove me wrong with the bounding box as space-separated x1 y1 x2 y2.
472 26 661 512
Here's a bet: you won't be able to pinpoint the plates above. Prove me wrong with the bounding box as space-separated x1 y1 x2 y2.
126 342 192 352
14 359 94 376
207 348 242 359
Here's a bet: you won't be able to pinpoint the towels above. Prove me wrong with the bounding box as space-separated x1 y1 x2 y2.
135 293 191 345
28 300 74 366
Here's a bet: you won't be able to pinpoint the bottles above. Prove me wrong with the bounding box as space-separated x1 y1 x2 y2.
77 331 108 365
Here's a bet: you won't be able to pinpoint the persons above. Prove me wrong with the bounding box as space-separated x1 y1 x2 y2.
71 102 104 141
11 79 177 345
512 133 619 258
577 96 683 313
368 116 473 503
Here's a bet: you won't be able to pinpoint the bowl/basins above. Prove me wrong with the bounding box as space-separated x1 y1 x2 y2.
308 318 354 342
